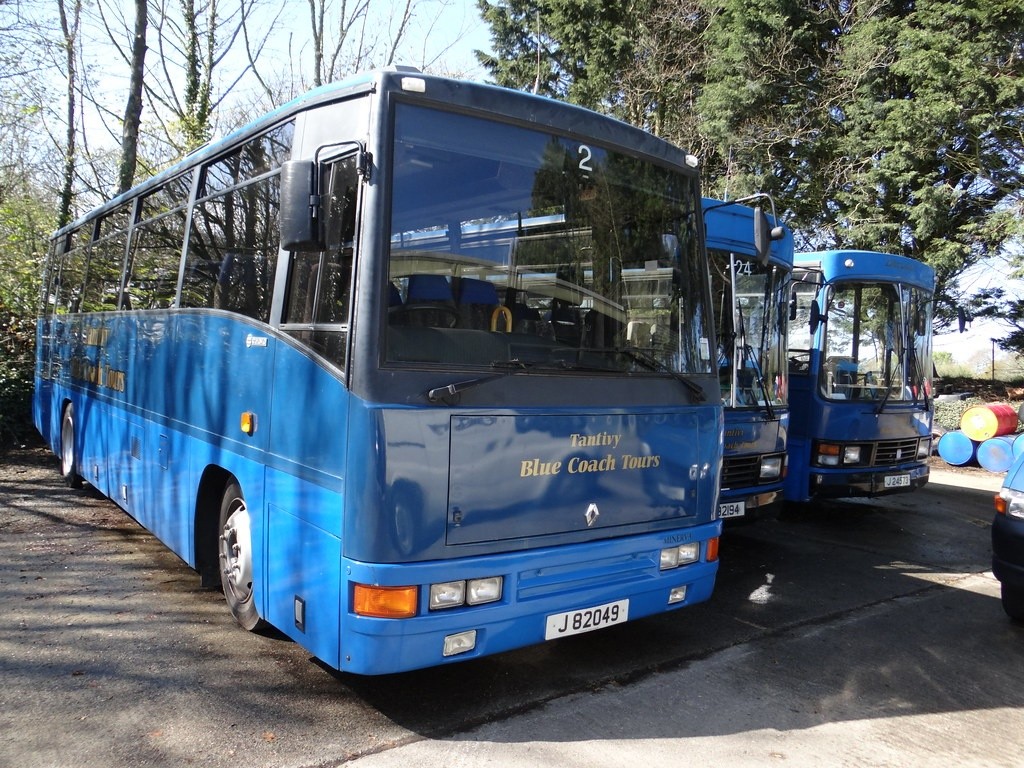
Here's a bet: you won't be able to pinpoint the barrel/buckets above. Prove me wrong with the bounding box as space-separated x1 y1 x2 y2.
976 434 1020 473
960 404 1018 441
1012 432 1024 460
938 429 981 466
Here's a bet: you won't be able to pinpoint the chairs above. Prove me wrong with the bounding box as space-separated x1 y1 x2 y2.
188 249 349 366
452 272 505 333
401 274 447 315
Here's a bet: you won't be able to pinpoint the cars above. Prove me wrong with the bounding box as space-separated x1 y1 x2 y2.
991 451 1024 620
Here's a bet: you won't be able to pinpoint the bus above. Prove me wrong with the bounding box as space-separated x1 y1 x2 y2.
791 245 973 500
28 65 785 680
701 194 834 518
